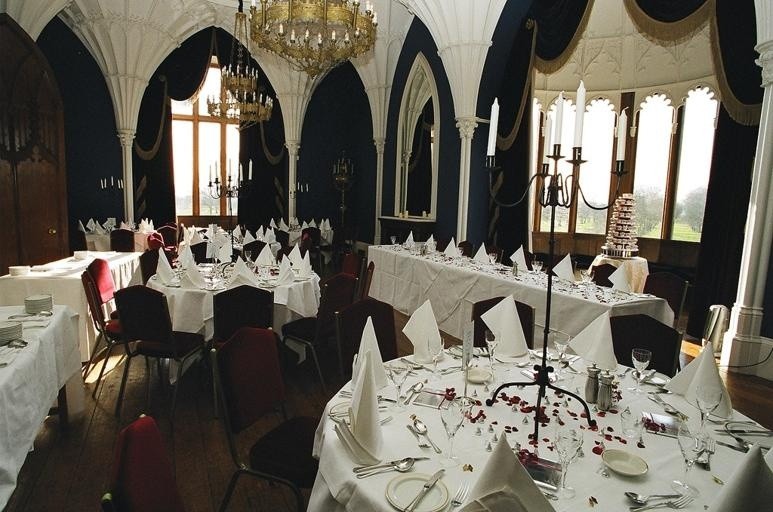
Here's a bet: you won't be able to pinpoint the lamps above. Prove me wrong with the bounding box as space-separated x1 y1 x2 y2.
246 0 380 78
205 0 273 130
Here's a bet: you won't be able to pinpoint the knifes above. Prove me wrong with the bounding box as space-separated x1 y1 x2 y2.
406 467 445 511
352 456 429 472
378 397 396 402
401 357 433 372
713 428 772 434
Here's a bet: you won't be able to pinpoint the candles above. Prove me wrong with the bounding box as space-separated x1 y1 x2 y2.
553 90 564 144
99 175 123 190
615 109 627 160
286 181 309 196
571 79 587 147
208 159 252 182
486 97 500 156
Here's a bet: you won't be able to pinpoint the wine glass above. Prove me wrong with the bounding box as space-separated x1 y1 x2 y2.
389 364 410 408
484 331 499 370
389 235 597 297
154 250 311 291
428 336 444 374
549 331 571 376
671 422 707 498
550 428 582 499
439 399 464 460
627 348 651 394
693 385 721 440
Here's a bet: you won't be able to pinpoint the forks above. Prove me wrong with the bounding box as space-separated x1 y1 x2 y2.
445 482 468 511
627 496 693 512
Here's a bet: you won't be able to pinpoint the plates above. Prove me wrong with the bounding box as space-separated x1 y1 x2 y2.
0 293 53 346
462 369 491 384
601 448 649 478
631 370 672 387
8 265 52 278
448 345 480 358
533 348 561 360
725 420 772 448
330 402 392 428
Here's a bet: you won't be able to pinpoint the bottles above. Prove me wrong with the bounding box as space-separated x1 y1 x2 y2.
596 369 615 411
585 363 599 404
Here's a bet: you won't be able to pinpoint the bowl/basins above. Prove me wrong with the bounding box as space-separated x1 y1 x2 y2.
73 251 87 260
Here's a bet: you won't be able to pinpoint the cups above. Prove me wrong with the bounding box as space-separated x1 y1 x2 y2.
621 409 646 443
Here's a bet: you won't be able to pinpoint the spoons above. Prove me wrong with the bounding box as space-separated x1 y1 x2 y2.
413 418 441 455
404 381 423 405
357 458 414 479
623 490 682 504
651 392 677 414
